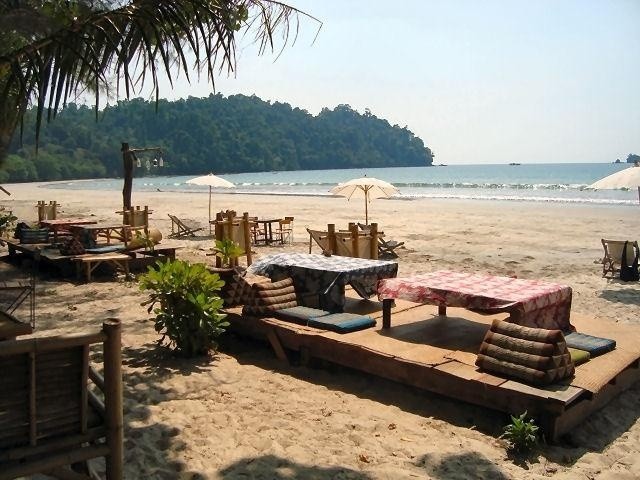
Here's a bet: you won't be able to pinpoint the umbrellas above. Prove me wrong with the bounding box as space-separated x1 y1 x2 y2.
183 173 235 220
583 166 640 190
327 175 403 225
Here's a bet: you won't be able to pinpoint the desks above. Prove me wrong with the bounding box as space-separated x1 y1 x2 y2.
71 224 130 246
246 253 398 312
35 218 97 243
377 270 576 333
0 279 36 330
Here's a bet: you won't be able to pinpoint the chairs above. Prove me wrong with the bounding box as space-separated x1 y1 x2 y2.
601 239 640 278
168 214 203 239
0 317 124 480
208 212 294 245
358 223 404 257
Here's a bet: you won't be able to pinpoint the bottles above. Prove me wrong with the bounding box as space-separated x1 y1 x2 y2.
159 156 164 167
145 159 151 168
136 157 142 168
152 158 158 167
